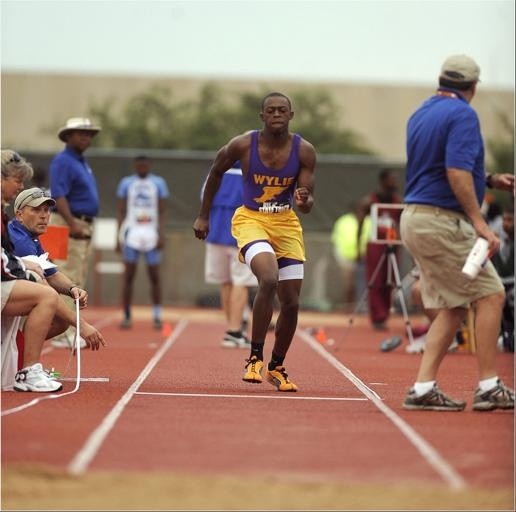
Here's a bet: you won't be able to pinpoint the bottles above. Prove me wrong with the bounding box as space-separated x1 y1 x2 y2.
377 211 397 241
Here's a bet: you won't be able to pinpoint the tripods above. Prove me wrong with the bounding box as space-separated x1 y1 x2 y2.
346 244 418 346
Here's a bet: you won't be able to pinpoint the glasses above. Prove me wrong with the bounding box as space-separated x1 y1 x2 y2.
7 152 22 164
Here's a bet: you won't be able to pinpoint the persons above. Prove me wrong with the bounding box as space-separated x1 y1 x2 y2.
241 287 278 336
330 169 515 357
191 91 316 392
48 116 103 351
8 187 108 369
199 158 252 349
399 57 515 413
1 149 63 393
114 155 170 331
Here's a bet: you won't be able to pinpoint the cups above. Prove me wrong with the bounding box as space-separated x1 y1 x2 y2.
461 237 493 281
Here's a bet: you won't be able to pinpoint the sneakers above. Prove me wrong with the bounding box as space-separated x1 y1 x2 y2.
12 362 63 394
401 382 466 412
241 355 265 384
224 329 251 348
471 377 516 411
264 362 298 392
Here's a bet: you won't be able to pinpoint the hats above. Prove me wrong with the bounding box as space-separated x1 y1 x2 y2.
438 53 484 84
57 116 101 143
12 186 56 214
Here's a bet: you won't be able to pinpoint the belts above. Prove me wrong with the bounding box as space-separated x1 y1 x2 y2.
403 202 468 221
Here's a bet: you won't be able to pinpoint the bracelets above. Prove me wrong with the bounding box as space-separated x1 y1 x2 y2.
68 284 77 297
